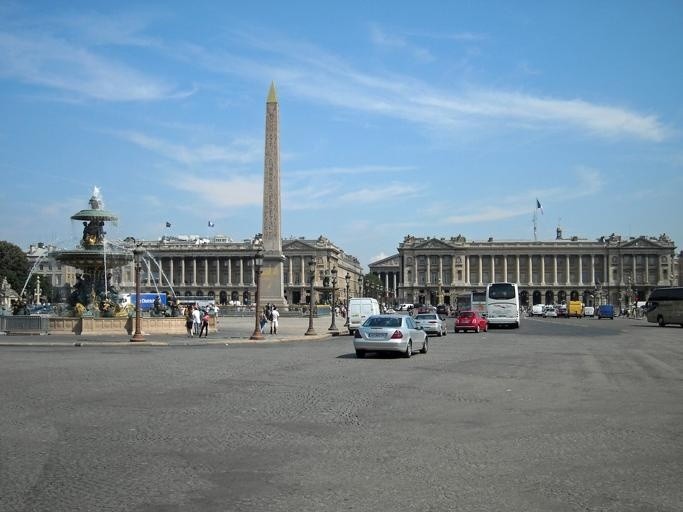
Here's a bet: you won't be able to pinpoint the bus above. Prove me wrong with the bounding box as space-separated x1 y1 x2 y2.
166 295 215 308
454 291 485 316
485 282 519 329
642 286 683 328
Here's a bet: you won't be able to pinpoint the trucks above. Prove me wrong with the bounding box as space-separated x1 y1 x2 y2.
347 297 382 335
116 292 166 311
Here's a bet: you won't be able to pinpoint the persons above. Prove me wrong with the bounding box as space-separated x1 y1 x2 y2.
334 300 347 319
186 302 220 338
259 303 280 334
520 305 637 320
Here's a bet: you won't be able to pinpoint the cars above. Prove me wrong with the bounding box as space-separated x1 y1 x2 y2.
412 314 447 337
378 302 457 317
27 304 56 314
530 301 595 318
353 314 428 358
454 311 488 333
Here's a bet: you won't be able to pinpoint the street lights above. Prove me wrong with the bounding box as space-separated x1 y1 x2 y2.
365 280 395 309
249 248 265 341
129 242 147 342
357 276 361 297
327 265 338 330
303 257 316 336
34 275 43 305
343 272 351 327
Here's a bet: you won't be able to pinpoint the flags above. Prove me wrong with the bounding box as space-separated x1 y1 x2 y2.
208 221 214 227
537 200 544 216
166 222 171 228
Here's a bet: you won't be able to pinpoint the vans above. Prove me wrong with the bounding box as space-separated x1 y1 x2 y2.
226 301 240 307
597 305 614 320
